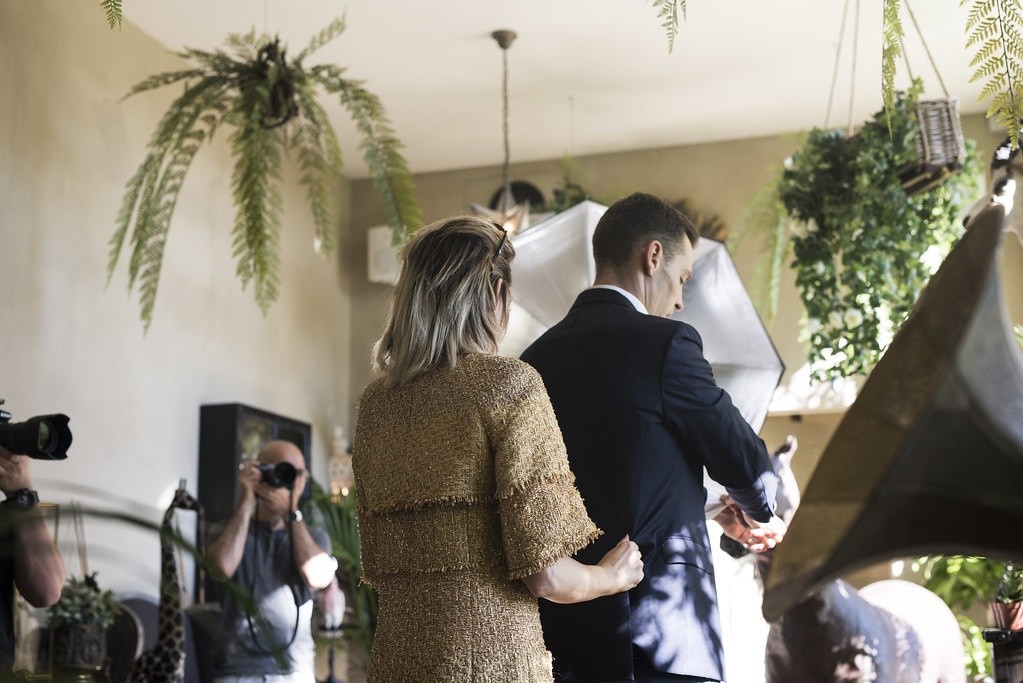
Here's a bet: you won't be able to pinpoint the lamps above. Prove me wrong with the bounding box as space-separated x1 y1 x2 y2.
469 30 555 235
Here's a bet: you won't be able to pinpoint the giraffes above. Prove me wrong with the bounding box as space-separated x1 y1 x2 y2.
129 479 200 683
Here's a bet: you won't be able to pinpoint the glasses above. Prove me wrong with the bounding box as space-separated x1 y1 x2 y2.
490 223 507 263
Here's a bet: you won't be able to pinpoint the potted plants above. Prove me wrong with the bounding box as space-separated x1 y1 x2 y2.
109 13 422 336
777 0 987 389
40 574 122 671
992 567 1023 631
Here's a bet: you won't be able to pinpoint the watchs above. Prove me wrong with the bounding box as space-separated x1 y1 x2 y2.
6 488 39 510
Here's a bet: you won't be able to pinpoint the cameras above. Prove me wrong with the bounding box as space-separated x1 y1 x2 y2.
256 462 297 497
0 409 73 461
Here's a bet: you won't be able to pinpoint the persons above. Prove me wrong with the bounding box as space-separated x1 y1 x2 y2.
0 454 66 683
520 190 965 683
207 439 338 683
352 215 644 683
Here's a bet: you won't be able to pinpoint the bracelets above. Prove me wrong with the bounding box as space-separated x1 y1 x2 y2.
284 511 302 524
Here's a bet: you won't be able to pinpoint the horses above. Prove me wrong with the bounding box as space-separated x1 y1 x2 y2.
719 434 967 683
